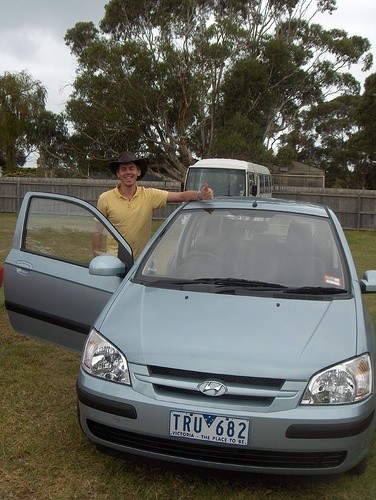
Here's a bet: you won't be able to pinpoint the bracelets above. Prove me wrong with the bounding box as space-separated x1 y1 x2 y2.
197 192 203 201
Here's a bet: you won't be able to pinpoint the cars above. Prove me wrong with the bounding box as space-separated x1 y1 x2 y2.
3 192 376 475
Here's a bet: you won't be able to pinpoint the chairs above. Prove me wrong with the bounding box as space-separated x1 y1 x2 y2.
176 223 322 287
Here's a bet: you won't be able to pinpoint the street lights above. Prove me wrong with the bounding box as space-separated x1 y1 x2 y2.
86 152 93 177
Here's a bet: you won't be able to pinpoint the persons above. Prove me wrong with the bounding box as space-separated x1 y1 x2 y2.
92 151 214 261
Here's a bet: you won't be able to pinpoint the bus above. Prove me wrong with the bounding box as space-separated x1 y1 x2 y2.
181 159 273 242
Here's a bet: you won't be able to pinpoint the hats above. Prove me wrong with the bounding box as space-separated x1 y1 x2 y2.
109 151 147 181
